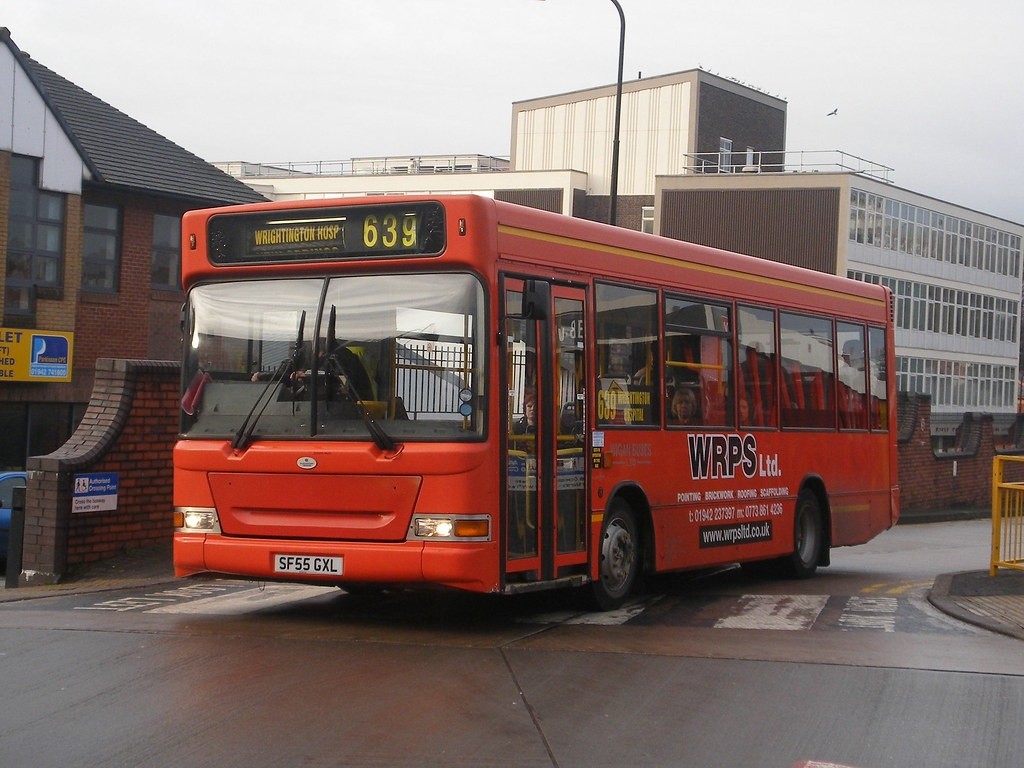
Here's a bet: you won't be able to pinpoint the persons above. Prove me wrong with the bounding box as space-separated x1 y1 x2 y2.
668 387 704 426
251 339 374 403
512 394 538 459
737 394 756 428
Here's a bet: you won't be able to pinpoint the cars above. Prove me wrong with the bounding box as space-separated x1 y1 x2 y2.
0 470 29 568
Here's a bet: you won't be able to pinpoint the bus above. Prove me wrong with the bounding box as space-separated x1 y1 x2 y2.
170 190 900 611
